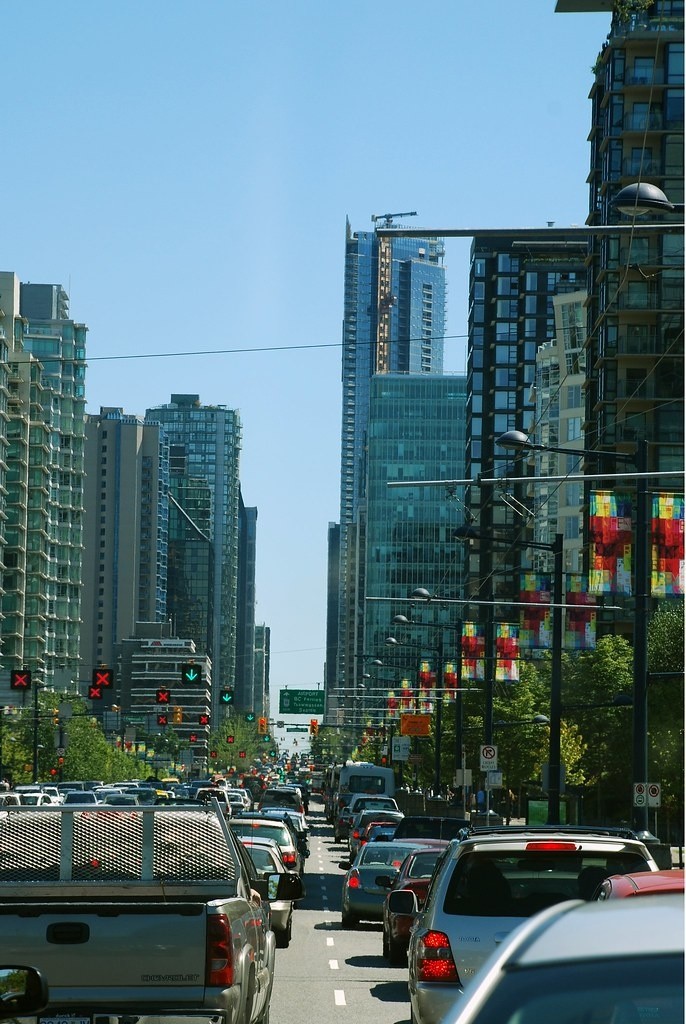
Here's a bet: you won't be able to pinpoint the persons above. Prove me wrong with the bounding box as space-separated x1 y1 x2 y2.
449 785 517 826
0 777 22 808
268 881 276 899
250 889 261 907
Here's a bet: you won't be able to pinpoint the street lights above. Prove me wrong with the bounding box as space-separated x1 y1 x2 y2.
496 427 648 833
450 526 634 826
20 665 45 709
346 587 493 822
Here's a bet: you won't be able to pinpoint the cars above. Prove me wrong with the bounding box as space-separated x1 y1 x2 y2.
285 751 684 1024
0 756 309 946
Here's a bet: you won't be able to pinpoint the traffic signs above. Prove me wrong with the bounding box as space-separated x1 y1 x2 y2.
278 689 326 714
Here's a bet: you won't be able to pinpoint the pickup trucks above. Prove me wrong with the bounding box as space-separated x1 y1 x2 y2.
0 794 309 1024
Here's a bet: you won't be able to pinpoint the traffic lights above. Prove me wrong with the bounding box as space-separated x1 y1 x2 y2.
258 718 266 735
310 719 319 735
10 668 32 691
91 668 113 688
50 767 58 775
182 663 202 684
88 685 104 701
158 708 284 774
155 689 171 702
219 690 236 705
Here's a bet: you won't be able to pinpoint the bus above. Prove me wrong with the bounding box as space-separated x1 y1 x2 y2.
332 761 396 843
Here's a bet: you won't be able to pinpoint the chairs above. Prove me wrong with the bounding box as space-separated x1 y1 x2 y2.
358 802 368 813
414 863 435 876
383 804 389 808
577 864 610 903
364 849 389 865
456 861 513 902
